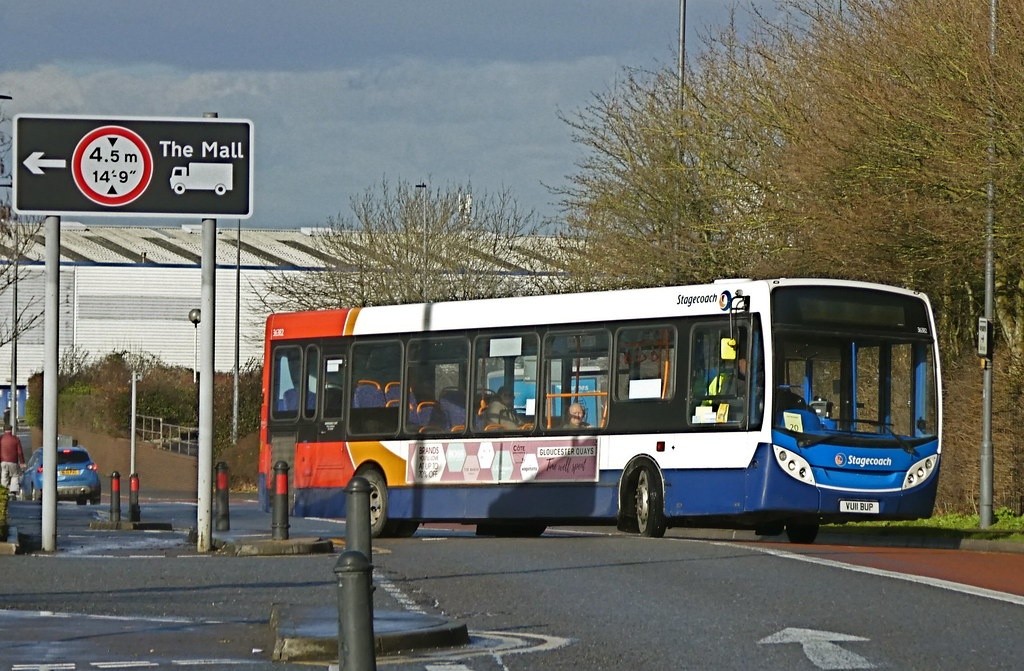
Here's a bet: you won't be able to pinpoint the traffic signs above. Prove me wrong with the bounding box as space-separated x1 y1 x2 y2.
977 317 989 358
12 113 255 219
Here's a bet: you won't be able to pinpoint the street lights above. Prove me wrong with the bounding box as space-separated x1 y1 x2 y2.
188 309 202 385
415 182 428 301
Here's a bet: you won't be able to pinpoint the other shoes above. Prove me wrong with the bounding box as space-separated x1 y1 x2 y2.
11 493 16 501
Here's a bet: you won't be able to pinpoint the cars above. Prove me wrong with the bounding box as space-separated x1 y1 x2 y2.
18 445 101 505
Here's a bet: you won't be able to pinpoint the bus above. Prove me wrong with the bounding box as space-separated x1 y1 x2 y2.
259 276 944 544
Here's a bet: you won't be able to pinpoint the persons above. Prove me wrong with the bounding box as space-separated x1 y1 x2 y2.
0 424 25 501
560 403 591 429
485 386 531 430
701 343 763 421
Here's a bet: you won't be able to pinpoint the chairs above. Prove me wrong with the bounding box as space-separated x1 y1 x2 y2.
284 379 501 433
